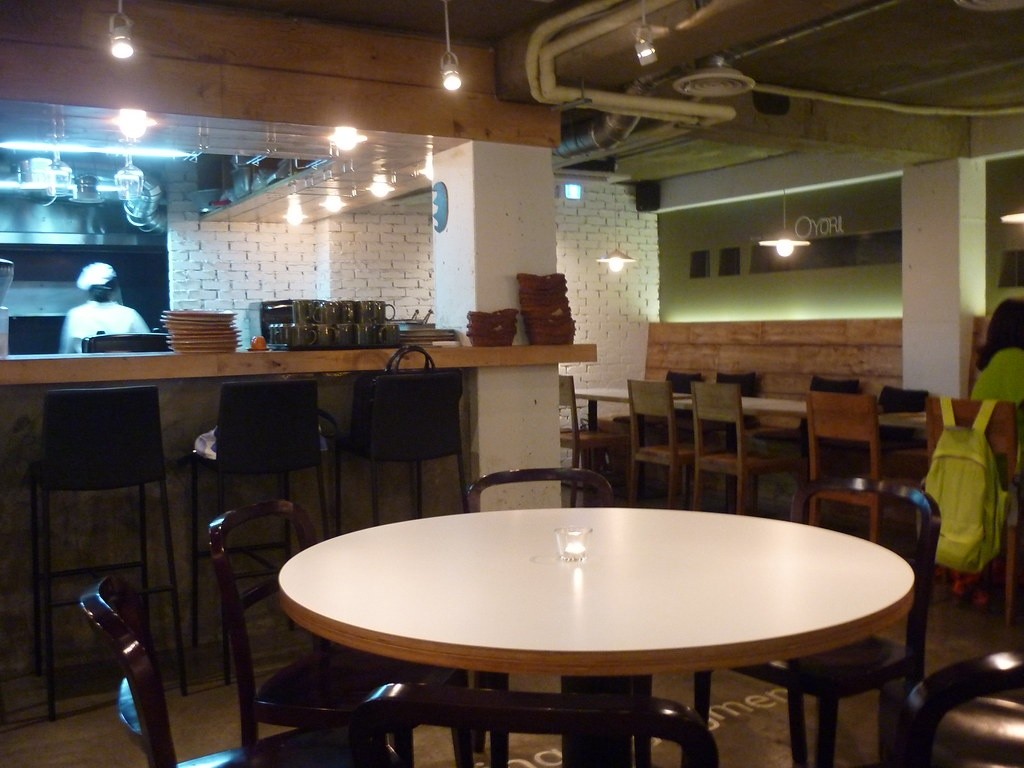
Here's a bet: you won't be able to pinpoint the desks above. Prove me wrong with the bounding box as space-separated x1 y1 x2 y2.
574 387 693 499
880 411 929 429
278 508 914 768
672 395 879 431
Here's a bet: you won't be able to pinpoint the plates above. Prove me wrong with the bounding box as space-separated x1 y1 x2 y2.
466 273 576 347
160 309 243 354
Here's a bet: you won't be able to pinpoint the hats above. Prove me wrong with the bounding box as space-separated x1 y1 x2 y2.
76 262 116 292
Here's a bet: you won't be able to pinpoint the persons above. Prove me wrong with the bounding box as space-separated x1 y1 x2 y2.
969 298 1024 499
58 262 151 354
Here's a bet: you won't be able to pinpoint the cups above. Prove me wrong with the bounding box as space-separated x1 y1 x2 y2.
556 526 593 561
269 299 400 349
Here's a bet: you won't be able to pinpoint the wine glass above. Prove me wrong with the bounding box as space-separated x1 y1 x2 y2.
115 141 144 200
46 135 72 197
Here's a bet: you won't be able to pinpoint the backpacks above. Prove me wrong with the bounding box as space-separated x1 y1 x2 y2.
921 395 1024 575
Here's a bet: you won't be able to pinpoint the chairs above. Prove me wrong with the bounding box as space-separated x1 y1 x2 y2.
207 498 474 768
29 384 190 723
193 378 342 687
553 366 1024 768
79 575 362 768
335 370 470 541
464 467 615 755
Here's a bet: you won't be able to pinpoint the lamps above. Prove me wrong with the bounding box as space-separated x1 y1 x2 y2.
757 153 812 258
439 0 462 91
633 0 658 66
110 0 134 59
596 184 636 272
